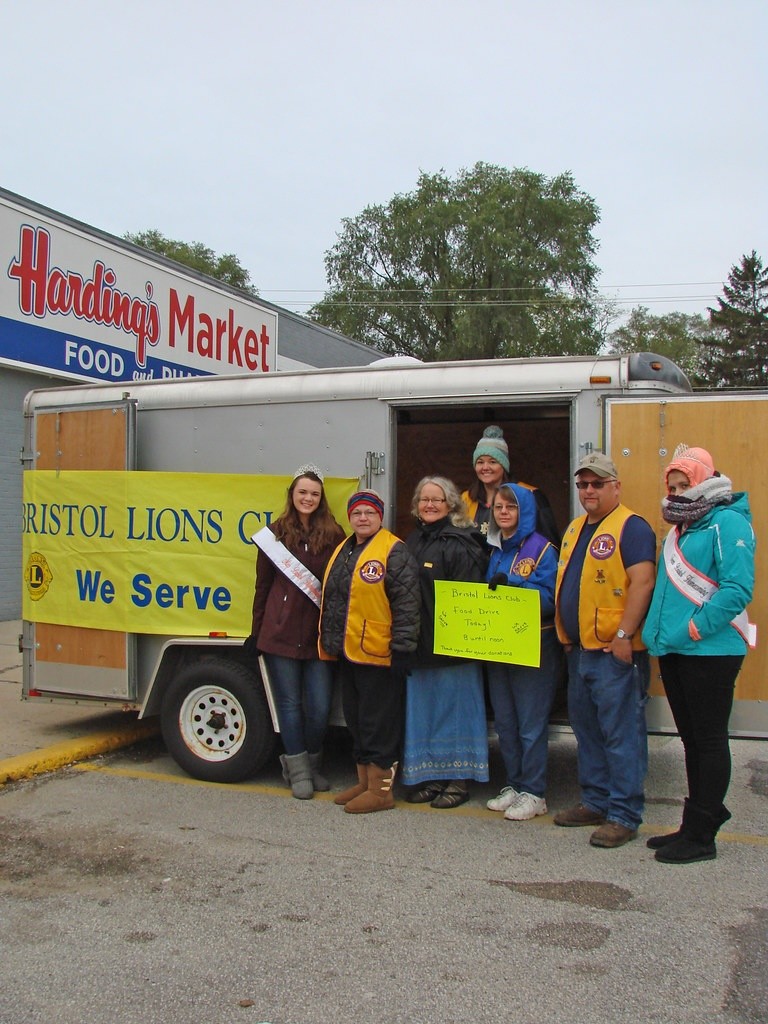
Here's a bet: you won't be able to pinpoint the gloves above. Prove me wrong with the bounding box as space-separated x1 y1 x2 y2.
244 634 261 658
487 572 509 590
389 652 412 692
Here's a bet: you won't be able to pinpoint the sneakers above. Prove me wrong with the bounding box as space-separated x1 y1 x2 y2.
430 787 472 808
503 792 548 820
554 800 606 827
486 786 518 811
407 784 441 802
589 820 637 848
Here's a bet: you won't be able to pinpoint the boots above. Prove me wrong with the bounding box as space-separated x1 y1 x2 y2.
279 750 313 799
334 763 369 805
344 762 398 813
307 747 330 792
655 797 720 863
647 803 732 851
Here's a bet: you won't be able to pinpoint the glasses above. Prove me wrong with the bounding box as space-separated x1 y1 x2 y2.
490 503 519 511
419 497 445 507
576 480 617 489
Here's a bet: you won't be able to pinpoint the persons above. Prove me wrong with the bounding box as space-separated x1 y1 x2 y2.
555 452 656 847
459 425 561 555
318 490 421 814
642 446 755 864
485 483 559 821
245 463 347 800
401 477 489 808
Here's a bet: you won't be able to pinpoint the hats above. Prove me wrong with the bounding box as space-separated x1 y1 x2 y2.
472 426 510 474
665 446 714 487
573 453 618 481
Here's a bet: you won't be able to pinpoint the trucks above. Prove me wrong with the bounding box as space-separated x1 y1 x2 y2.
19 351 768 784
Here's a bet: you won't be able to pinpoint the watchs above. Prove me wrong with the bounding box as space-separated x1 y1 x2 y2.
616 628 635 641
